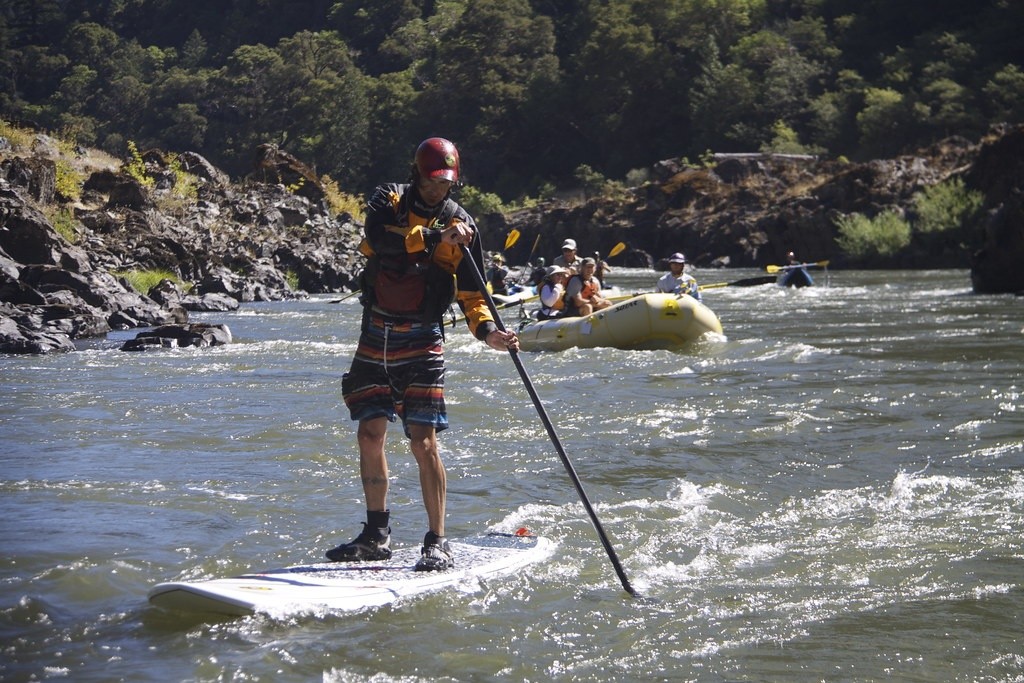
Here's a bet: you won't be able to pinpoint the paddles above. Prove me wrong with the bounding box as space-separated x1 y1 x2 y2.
457 242 635 595
443 317 465 326
519 234 540 284
766 260 829 274
329 289 362 303
603 242 626 261
605 276 778 300
487 229 521 274
496 294 540 310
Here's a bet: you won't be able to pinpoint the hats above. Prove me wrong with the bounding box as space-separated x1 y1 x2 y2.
493 255 503 261
581 258 597 266
561 239 577 250
545 265 567 277
668 253 686 264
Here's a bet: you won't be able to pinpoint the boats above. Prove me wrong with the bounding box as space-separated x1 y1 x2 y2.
507 289 724 355
780 266 816 289
487 284 622 316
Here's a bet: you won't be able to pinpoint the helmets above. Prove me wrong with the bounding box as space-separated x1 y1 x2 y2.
414 137 460 184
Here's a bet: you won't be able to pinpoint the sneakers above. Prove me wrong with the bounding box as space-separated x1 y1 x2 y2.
416 530 454 571
325 522 392 562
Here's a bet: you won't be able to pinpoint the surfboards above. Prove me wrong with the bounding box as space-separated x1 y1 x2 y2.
147 531 554 617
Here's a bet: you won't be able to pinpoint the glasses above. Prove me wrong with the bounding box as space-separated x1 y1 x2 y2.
562 248 575 253
552 273 564 277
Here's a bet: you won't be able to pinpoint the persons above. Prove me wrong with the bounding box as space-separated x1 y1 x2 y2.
524 257 546 286
553 238 584 276
536 265 570 320
655 252 702 302
775 251 806 284
485 254 520 303
563 257 611 318
324 137 520 574
592 250 611 286
